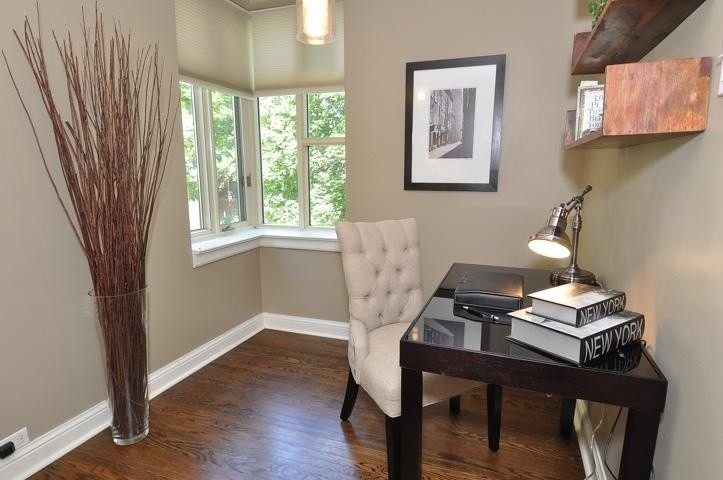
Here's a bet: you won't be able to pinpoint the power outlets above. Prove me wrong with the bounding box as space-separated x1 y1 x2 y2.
0 426 30 450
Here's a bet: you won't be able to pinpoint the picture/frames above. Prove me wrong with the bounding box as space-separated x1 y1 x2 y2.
404 53 505 192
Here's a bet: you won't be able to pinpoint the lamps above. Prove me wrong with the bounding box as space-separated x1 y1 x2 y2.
528 186 595 285
295 0 335 44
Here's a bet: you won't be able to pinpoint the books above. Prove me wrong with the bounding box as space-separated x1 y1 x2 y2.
505 310 644 367
507 341 641 374
573 80 605 141
525 284 624 329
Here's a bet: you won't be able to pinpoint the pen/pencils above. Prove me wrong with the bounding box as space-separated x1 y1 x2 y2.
460 305 499 321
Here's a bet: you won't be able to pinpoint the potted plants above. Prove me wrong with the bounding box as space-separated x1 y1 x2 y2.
2 2 181 446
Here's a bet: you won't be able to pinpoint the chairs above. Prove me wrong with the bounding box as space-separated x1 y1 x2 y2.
335 218 503 480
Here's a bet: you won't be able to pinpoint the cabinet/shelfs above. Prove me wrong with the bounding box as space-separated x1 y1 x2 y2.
564 0 710 150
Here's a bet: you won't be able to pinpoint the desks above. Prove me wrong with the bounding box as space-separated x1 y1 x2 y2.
399 261 668 480
